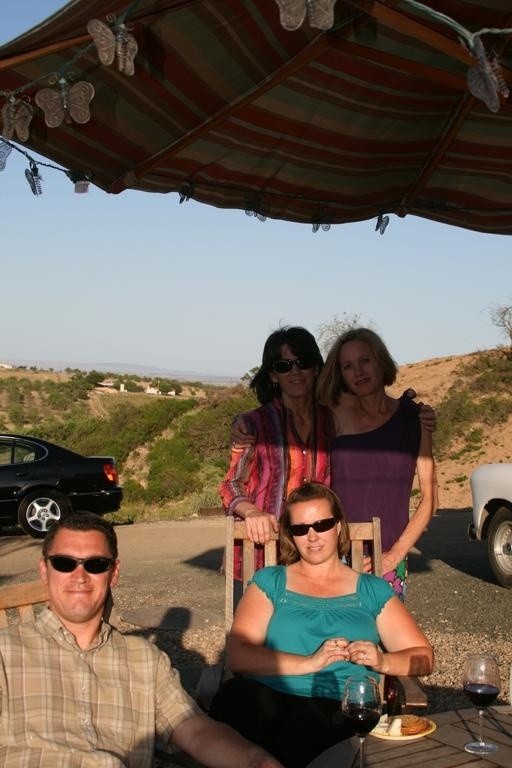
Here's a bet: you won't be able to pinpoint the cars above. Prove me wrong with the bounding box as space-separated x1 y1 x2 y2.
0 434 124 538
466 463 512 588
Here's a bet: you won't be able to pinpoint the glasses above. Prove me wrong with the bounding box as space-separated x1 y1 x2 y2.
272 357 315 374
45 554 115 575
286 516 340 537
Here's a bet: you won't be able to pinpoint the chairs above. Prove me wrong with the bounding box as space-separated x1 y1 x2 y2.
195 515 382 713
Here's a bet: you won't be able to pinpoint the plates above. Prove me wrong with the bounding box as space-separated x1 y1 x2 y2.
368 713 438 743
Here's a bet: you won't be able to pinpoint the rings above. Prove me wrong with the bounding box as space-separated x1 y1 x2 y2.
334 639 338 646
232 441 235 448
363 651 367 660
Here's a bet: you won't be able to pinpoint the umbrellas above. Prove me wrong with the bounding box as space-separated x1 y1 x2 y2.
0 1 510 238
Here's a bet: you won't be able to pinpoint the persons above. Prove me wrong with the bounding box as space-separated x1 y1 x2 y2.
207 482 436 767
1 508 288 768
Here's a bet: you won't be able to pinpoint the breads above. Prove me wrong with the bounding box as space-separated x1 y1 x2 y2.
390 714 428 736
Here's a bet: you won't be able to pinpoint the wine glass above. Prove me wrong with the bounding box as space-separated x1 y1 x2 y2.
339 673 384 768
462 652 502 756
